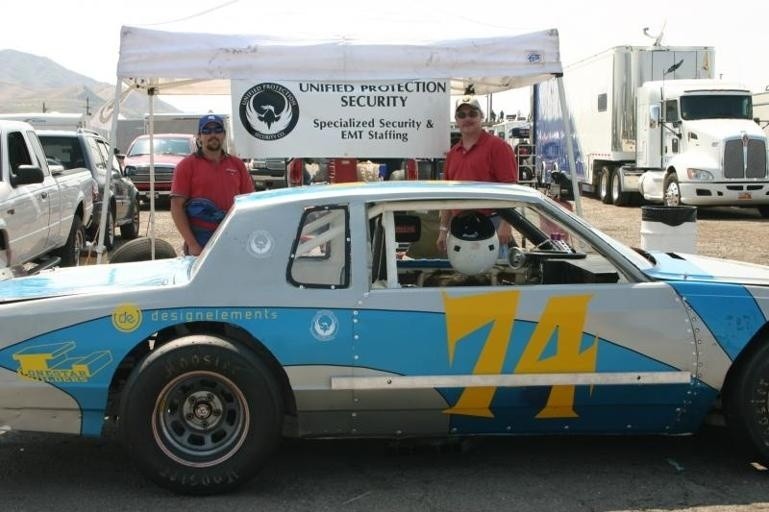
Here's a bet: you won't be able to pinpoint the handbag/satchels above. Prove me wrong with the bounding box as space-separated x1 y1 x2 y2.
184 198 227 249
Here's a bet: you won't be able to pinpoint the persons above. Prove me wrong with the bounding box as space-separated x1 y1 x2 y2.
169 114 256 257
434 97 519 260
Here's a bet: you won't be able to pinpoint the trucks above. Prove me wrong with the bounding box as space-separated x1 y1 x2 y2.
531 45 769 219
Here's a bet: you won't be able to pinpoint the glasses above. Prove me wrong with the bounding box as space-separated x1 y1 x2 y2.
201 126 224 134
456 111 478 119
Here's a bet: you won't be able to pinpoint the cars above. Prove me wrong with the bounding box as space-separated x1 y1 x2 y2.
0 179 769 497
249 159 288 191
0 120 197 267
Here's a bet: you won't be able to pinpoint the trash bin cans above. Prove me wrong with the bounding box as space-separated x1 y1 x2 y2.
640 206 698 255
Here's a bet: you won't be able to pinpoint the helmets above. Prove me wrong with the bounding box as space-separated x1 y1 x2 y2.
519 167 532 180
446 209 501 276
514 142 532 161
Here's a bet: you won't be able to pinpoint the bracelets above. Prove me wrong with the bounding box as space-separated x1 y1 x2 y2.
439 224 450 231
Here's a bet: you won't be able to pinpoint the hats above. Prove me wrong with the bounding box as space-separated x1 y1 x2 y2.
198 114 224 135
454 95 482 115
